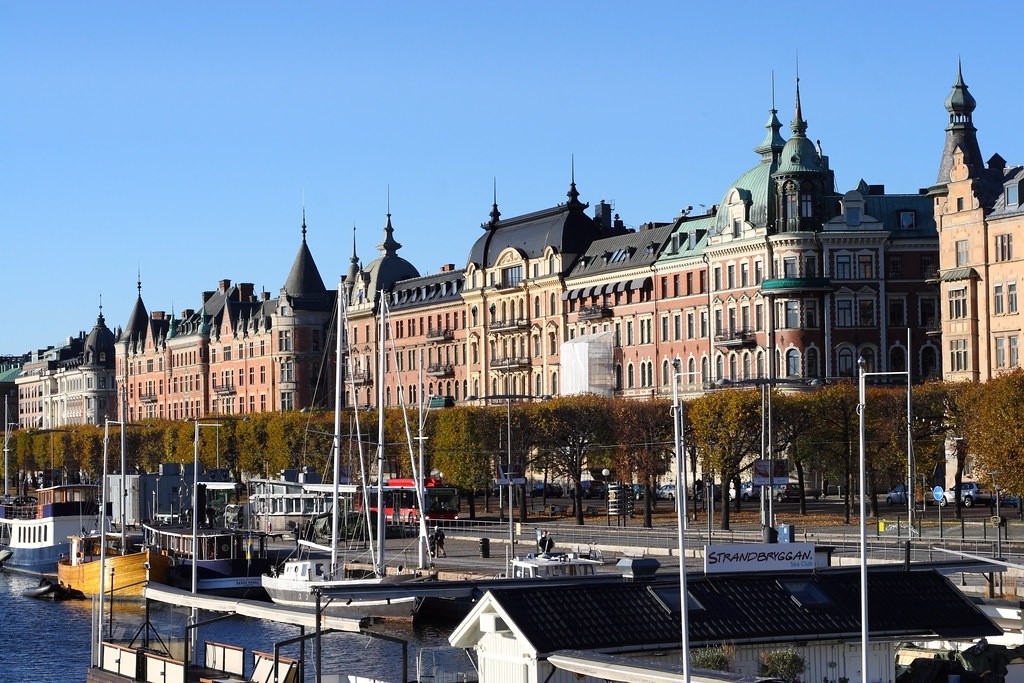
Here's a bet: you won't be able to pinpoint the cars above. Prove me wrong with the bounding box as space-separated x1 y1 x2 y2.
529 483 563 499
993 494 1022 509
884 480 934 506
696 484 725 502
655 483 676 501
12 496 38 511
599 482 646 501
570 478 604 500
760 481 802 504
940 480 995 508
728 480 759 502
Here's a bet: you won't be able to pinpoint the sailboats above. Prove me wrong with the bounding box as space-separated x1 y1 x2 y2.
147 282 430 620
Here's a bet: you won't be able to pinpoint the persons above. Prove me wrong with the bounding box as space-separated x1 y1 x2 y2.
206 504 218 530
425 525 447 557
695 478 703 501
539 530 553 553
294 520 314 551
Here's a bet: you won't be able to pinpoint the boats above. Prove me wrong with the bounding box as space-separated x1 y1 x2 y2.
0 485 109 577
56 526 175 603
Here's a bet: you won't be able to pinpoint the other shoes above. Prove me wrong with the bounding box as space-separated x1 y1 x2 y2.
443 553 446 558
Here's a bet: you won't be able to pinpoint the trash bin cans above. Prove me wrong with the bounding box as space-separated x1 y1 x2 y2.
478 537 490 558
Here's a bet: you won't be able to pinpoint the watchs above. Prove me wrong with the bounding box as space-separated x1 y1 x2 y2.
823 478 829 498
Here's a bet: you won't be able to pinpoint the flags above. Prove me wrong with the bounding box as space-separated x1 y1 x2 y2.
247 530 255 564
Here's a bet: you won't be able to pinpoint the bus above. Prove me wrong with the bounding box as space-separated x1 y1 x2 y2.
356 478 461 529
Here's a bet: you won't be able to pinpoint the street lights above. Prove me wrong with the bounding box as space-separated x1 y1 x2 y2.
464 393 553 565
98 413 155 671
714 374 824 542
182 416 250 481
298 406 376 484
667 353 707 683
601 468 611 526
855 355 874 683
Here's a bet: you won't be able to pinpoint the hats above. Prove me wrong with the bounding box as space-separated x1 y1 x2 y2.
434 526 438 530
